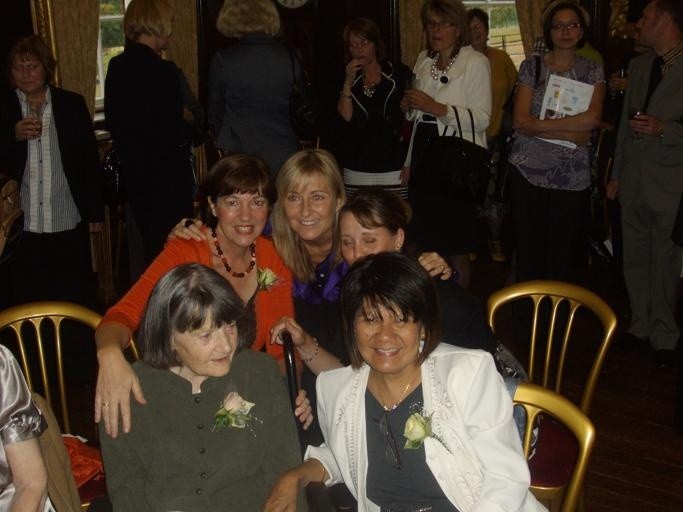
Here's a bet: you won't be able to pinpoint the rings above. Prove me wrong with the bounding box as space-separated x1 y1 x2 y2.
100 401 111 410
183 218 195 229
438 264 447 274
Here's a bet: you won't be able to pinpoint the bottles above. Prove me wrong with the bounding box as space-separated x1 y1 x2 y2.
98 139 120 178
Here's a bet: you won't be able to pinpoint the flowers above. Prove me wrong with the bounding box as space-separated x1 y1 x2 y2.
210 391 262 438
403 409 453 454
253 267 284 303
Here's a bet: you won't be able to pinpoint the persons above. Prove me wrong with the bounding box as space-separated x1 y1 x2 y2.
606 15 654 106
97 261 308 512
0 345 54 510
261 149 470 304
605 1 683 367
573 7 603 61
509 4 608 324
339 188 539 458
336 15 413 203
462 9 518 264
105 0 205 261
92 152 315 432
1 34 102 311
262 252 548 512
199 1 315 164
399 0 494 251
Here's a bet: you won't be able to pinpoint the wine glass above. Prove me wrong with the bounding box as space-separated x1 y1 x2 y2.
405 80 420 119
628 109 647 143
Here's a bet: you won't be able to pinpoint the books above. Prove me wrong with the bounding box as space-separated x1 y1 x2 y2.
534 73 597 151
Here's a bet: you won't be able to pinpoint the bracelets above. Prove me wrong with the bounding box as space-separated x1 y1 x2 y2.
340 92 353 102
302 338 319 364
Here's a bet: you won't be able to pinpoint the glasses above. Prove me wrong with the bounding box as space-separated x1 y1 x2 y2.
551 22 582 29
374 411 402 469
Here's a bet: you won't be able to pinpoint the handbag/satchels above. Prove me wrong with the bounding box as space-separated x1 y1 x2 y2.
424 106 489 202
501 55 541 138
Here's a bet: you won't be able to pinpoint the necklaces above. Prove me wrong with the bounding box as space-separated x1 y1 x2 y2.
429 49 459 79
212 228 256 278
359 65 382 97
372 369 417 410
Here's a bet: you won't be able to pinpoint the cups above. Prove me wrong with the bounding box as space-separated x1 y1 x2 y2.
26 101 43 136
616 68 629 95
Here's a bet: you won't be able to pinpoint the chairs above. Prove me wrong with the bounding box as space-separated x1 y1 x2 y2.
487 280 618 512
587 122 618 264
512 382 596 512
190 128 223 217
0 301 139 512
